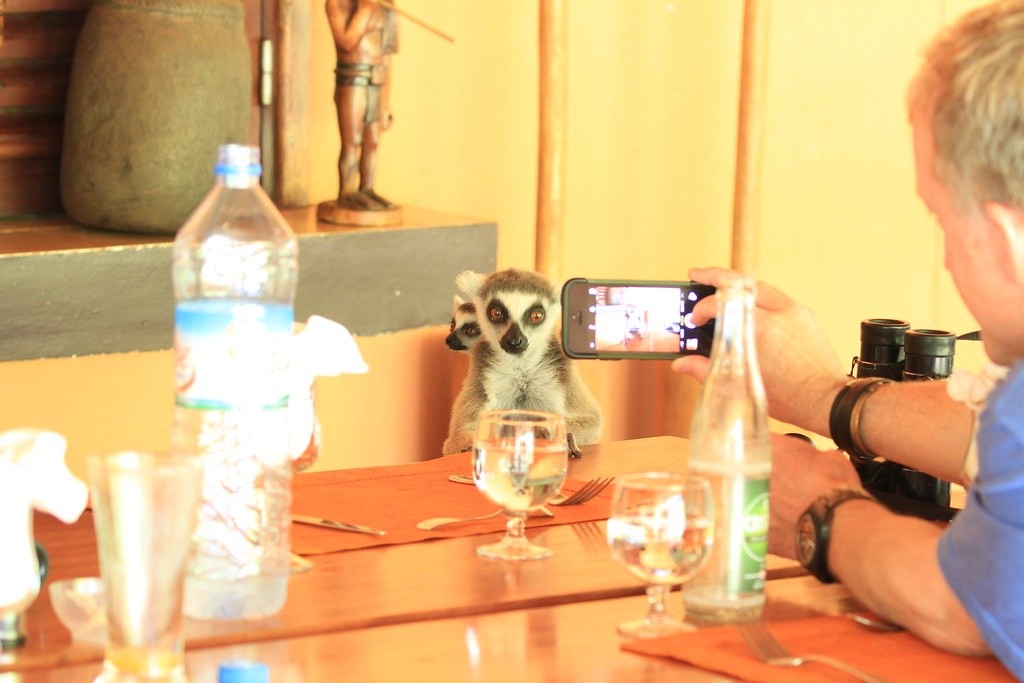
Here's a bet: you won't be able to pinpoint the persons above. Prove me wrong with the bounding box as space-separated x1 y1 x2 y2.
673 0 1024 683
326 0 400 213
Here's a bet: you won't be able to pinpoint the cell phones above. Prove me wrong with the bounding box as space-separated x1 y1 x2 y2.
562 277 717 361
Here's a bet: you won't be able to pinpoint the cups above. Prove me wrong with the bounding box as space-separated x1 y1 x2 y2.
47 576 107 642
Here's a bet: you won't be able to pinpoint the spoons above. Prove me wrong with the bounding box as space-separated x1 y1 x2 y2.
417 504 553 532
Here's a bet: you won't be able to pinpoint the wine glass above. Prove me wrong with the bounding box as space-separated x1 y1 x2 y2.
611 471 714 638
474 410 567 560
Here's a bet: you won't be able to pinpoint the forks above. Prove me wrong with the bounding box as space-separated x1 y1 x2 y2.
738 612 881 683
447 474 617 505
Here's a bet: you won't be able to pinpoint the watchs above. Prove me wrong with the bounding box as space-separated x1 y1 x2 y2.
794 490 883 584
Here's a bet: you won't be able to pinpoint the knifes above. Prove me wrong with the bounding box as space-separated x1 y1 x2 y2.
292 513 386 536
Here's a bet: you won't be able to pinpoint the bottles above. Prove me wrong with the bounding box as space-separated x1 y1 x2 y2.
166 144 302 620
85 444 195 683
687 275 772 621
218 660 271 683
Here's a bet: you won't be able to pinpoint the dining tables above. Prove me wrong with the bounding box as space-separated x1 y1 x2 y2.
0 434 959 683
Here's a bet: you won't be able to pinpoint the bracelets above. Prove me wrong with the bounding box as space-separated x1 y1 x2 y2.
829 377 897 462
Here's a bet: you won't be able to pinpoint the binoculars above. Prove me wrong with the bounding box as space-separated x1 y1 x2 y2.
848 318 958 523
